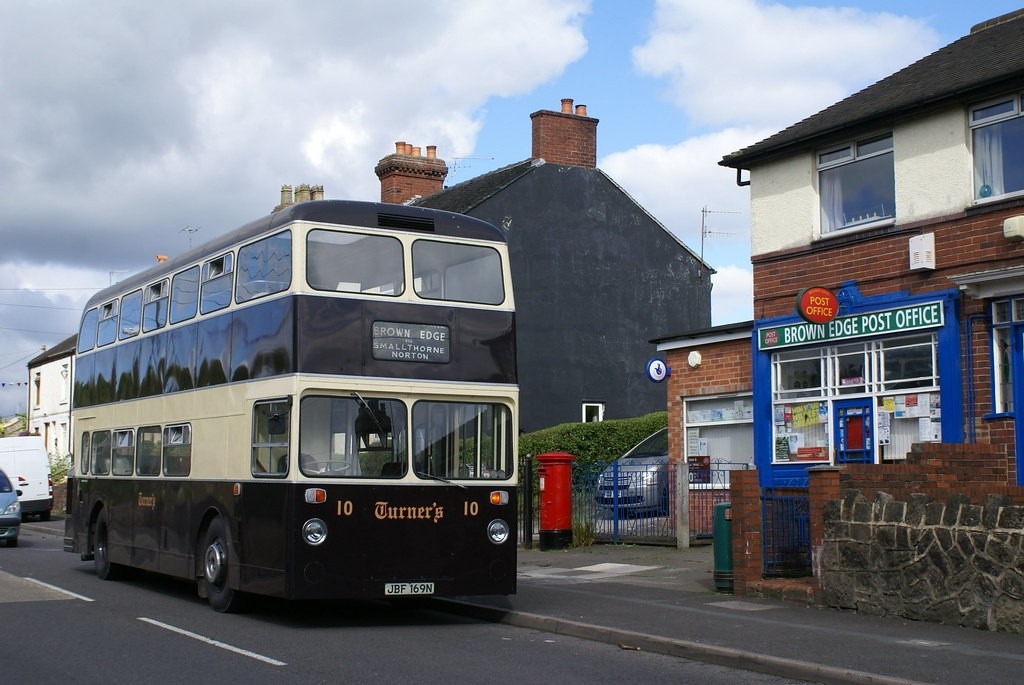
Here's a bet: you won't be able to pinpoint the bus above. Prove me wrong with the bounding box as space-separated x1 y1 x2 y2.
62 199 520 614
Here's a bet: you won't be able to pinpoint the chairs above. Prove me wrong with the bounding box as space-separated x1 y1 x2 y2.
278 453 319 475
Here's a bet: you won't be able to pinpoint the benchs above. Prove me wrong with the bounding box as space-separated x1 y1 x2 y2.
380 462 433 480
95 455 190 476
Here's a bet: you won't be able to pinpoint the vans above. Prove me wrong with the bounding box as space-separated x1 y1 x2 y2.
0 437 55 523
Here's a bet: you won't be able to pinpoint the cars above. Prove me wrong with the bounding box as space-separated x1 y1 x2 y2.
0 471 23 546
593 428 668 514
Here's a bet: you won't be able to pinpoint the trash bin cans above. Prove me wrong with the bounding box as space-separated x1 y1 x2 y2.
711 502 734 594
